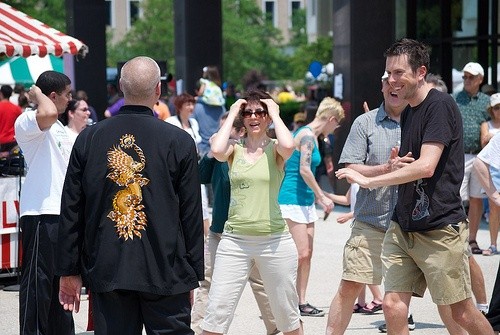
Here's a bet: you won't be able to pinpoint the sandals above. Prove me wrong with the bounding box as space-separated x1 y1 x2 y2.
352 303 367 314
361 300 382 313
469 240 483 254
483 246 500 256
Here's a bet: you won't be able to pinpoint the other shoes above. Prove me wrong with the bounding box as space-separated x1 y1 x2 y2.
379 313 416 333
299 301 325 316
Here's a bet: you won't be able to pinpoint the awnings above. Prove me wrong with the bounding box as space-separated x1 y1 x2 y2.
0 53 64 92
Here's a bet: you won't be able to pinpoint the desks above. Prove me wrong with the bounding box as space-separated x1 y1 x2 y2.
0 173 25 277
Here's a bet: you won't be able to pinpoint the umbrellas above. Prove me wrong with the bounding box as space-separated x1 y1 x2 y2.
0 2 89 67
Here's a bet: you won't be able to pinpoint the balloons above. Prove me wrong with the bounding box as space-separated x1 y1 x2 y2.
306 72 313 80
309 61 322 79
326 63 334 75
323 74 328 81
223 82 227 88
317 73 323 80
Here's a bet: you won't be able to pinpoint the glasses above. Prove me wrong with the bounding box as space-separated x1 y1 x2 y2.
242 110 268 119
461 75 475 80
79 107 89 112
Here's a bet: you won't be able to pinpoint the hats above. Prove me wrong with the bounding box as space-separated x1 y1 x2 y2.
490 93 500 107
462 62 484 76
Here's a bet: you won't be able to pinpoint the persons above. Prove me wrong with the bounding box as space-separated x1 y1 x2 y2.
198 88 305 335
277 97 345 317
449 62 500 335
321 182 383 313
58 97 91 294
14 70 75 335
325 70 408 335
334 37 495 335
0 66 343 238
55 55 205 335
190 111 283 335
363 74 489 332
